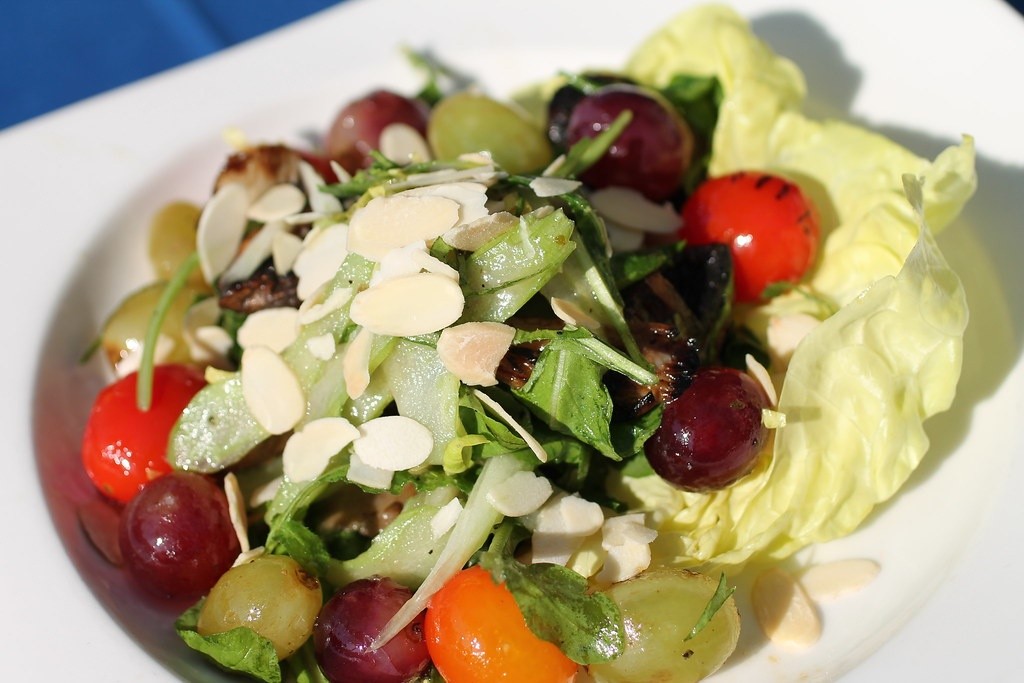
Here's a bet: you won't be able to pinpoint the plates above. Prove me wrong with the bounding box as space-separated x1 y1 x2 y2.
0 2 1019 683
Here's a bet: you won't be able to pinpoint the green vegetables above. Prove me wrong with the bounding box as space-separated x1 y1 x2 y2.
170 148 725 682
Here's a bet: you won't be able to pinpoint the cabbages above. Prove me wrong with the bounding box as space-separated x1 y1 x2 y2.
625 0 977 568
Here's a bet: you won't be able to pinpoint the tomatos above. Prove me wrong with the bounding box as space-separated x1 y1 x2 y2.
80 363 205 506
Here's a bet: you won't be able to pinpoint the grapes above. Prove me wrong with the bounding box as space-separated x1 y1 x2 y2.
118 471 239 610
326 89 431 171
584 570 742 683
565 84 694 195
100 280 202 374
424 92 553 176
